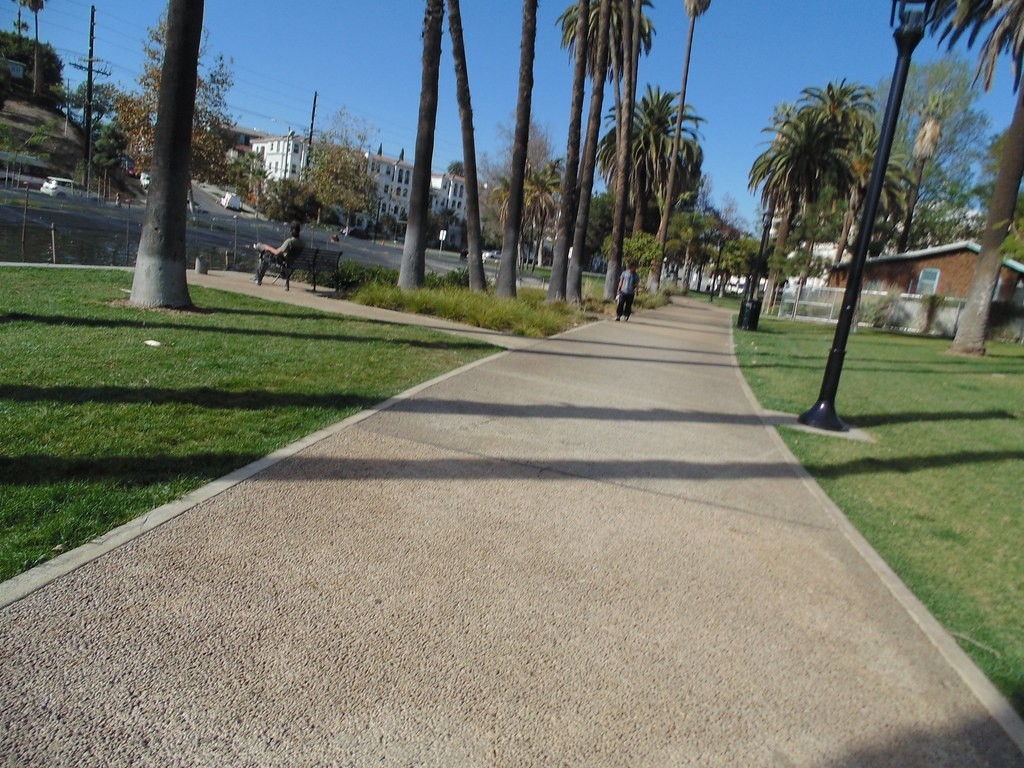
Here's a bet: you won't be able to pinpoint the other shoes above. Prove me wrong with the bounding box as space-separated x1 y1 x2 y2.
624 316 629 321
616 315 620 321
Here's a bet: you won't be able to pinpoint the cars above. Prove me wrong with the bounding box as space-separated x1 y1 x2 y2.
141 175 150 189
39 177 99 199
339 227 372 241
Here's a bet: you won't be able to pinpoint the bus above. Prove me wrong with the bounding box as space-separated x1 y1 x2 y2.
0 151 72 190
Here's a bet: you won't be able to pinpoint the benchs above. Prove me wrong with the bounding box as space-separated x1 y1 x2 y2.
255 244 343 292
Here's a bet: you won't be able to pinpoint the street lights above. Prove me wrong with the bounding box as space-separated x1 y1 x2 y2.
284 130 295 179
794 0 941 432
742 211 774 329
708 238 725 301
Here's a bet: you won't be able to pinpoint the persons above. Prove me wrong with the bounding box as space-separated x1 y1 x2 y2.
114 192 122 208
250 220 305 282
615 261 640 321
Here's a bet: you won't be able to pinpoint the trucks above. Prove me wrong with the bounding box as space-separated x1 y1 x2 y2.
220 192 241 211
